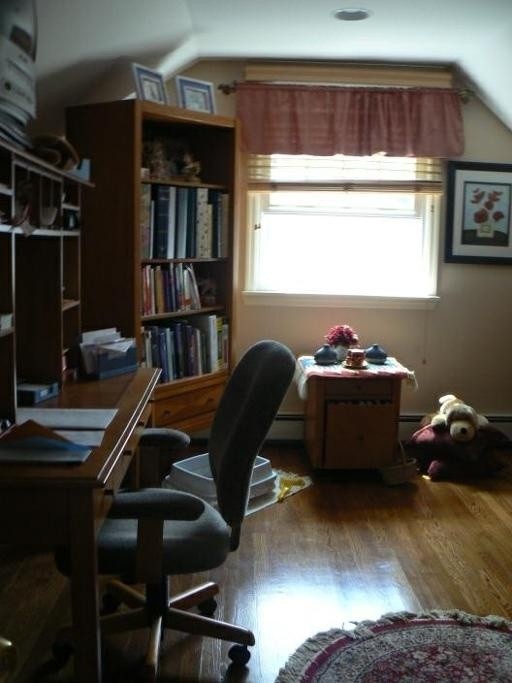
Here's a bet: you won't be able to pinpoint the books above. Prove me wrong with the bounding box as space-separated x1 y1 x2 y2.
137 179 232 386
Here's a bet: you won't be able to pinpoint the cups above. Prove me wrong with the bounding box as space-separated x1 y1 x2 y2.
347 349 364 365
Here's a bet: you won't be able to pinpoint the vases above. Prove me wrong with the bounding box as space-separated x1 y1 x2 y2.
335 344 349 361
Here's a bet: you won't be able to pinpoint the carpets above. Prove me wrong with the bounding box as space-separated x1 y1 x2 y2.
275 609 512 683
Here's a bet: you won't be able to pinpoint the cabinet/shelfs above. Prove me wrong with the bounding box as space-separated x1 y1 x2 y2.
64 101 243 436
0 141 95 444
294 357 419 476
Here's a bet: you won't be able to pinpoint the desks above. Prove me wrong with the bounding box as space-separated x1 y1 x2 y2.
0 367 165 683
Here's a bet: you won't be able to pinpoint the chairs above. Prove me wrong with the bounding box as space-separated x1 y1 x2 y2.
51 338 295 683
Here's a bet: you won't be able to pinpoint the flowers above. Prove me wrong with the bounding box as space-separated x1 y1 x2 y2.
324 324 360 348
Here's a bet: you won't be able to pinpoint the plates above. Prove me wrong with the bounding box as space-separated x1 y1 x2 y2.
342 360 369 370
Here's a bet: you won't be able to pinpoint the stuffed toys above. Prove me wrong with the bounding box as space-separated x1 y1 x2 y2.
401 392 511 485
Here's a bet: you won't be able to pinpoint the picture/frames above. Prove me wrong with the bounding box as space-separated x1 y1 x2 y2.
174 75 216 114
132 62 168 106
444 161 512 265
